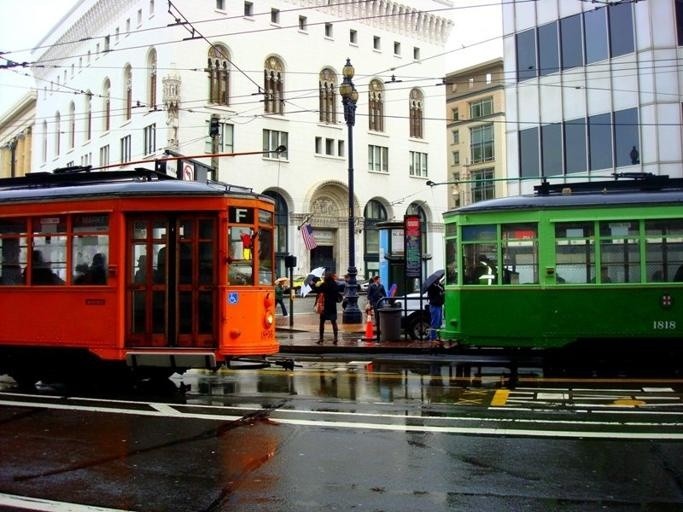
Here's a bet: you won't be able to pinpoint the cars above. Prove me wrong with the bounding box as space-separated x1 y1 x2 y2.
283 276 372 295
394 272 446 342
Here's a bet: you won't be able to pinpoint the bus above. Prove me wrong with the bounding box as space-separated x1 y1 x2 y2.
431 168 682 366
0 161 282 388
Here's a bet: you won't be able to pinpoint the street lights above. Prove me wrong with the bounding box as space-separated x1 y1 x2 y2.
339 57 362 324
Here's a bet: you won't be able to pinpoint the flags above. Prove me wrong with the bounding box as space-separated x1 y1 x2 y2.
299 222 317 250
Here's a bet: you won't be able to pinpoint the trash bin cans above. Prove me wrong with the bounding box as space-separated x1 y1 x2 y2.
377 308 403 343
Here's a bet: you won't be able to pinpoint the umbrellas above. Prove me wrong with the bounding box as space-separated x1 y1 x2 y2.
419 268 445 294
299 266 326 298
273 277 290 289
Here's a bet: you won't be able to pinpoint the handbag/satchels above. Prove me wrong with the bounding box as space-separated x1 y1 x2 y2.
334 281 344 303
312 283 326 314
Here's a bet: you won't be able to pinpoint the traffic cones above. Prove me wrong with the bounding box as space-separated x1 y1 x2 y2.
362 358 376 386
361 307 377 340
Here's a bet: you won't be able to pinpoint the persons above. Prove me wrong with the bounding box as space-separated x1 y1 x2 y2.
366 276 386 326
309 270 342 344
473 254 497 286
590 266 612 283
427 278 444 343
275 281 287 318
0 233 211 347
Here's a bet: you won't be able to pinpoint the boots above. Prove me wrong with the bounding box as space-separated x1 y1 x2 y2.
317 328 325 344
333 327 338 344
